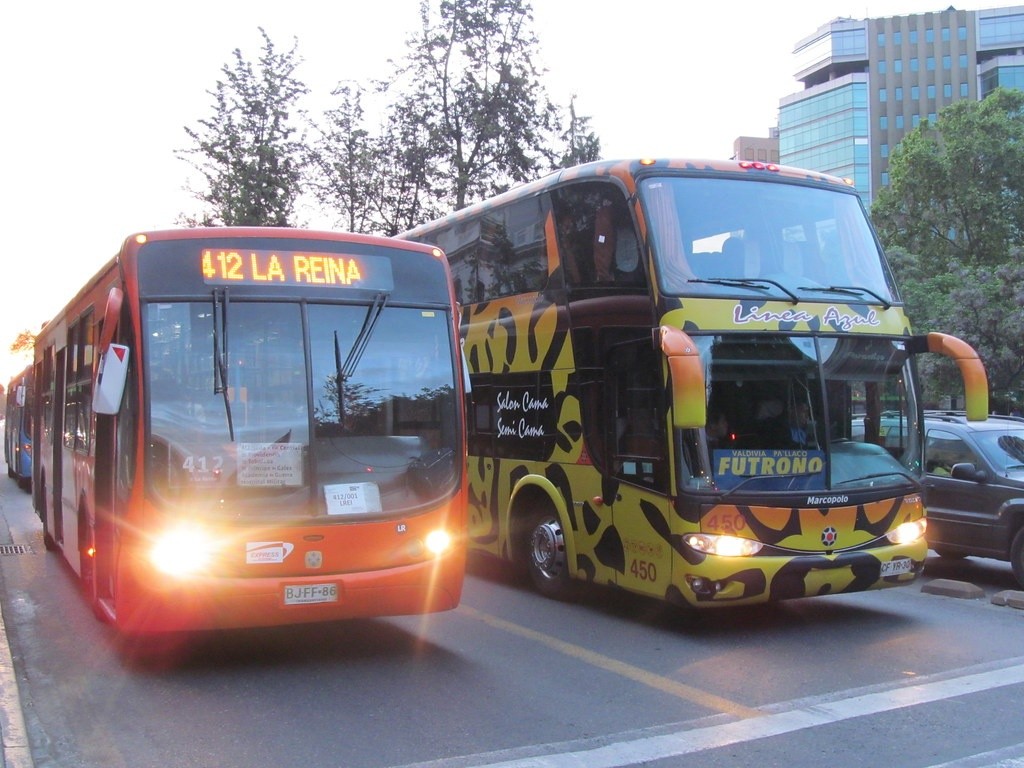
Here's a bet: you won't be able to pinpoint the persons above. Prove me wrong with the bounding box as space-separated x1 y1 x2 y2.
774 398 816 451
702 407 730 475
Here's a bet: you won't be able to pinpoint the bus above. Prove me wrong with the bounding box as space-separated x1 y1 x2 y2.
3 366 38 490
29 226 476 651
389 155 988 610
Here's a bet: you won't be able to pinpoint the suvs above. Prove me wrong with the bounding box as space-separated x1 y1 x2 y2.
846 408 1023 596
